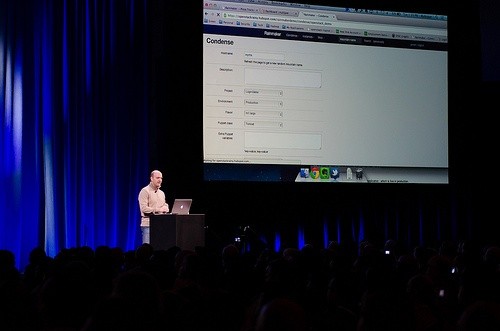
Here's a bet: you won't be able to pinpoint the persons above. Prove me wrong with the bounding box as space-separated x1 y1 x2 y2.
138 169 170 244
1 234 499 330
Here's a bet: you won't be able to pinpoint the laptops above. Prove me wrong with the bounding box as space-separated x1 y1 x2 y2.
169 198 192 215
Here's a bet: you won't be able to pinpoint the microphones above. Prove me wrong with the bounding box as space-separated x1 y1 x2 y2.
157 186 162 189
